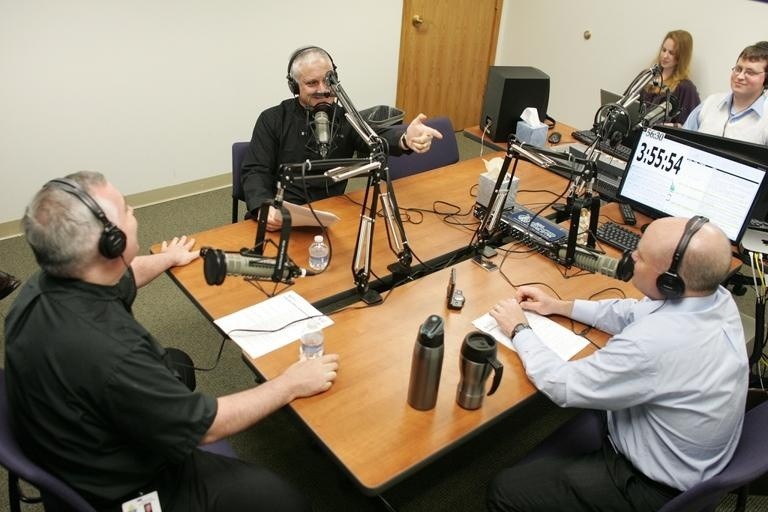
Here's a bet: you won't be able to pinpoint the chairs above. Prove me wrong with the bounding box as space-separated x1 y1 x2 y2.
366 114 461 183
230 139 251 223
506 392 768 511
2 367 253 512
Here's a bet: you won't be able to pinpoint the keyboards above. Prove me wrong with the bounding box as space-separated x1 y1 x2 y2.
572 130 632 162
595 221 642 252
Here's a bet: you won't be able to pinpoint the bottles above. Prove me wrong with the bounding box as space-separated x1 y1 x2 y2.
307 234 329 270
405 314 448 412
298 315 324 365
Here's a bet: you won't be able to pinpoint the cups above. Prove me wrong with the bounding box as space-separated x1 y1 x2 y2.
455 332 503 413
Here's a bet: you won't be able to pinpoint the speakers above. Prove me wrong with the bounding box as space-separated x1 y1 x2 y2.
480 65 549 142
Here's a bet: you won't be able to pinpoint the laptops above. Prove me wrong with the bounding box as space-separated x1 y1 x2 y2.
601 89 640 126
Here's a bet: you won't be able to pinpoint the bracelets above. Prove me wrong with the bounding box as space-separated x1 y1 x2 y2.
402 132 410 149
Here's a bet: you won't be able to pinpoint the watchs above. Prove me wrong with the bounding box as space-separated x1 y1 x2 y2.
510 324 534 342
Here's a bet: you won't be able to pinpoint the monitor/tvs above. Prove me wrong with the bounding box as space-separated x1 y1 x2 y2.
616 126 768 246
653 125 768 224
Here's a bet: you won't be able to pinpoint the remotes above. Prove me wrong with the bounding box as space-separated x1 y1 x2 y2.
619 203 637 225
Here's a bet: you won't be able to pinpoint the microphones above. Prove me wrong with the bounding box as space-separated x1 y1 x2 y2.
204 247 299 286
311 102 336 144
558 241 634 282
643 94 680 127
652 63 663 84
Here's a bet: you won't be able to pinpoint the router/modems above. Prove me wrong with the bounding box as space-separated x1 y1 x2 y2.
738 227 768 262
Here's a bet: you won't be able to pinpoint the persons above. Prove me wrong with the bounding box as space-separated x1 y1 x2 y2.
238 43 446 233
488 214 751 509
637 29 701 125
681 40 768 148
0 169 342 511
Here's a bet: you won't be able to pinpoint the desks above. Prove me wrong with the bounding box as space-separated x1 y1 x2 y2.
460 115 768 275
236 194 746 498
144 148 604 344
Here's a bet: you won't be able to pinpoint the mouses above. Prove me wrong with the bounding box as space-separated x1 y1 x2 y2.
548 130 561 143
641 224 650 233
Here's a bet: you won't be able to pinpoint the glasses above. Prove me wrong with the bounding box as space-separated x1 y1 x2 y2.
732 66 763 76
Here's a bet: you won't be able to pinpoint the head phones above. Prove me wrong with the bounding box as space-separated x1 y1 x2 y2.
287 47 338 96
656 215 709 299
593 103 631 147
43 177 127 259
